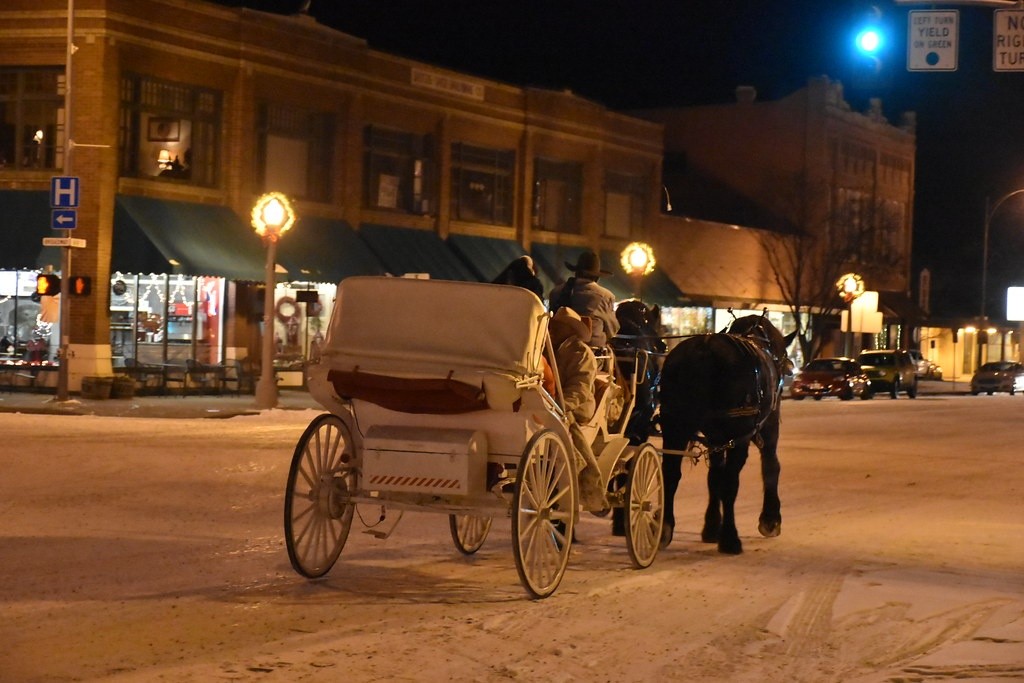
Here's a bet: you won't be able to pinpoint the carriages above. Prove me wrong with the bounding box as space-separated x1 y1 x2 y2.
283 270 803 600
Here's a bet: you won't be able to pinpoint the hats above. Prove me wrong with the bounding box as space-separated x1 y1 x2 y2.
565 251 614 278
548 306 590 343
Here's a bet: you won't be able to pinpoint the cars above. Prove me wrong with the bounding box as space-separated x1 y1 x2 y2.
790 356 874 401
915 360 942 380
970 361 1024 395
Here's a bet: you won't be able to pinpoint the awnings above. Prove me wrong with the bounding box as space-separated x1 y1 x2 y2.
110 193 684 304
878 291 926 325
0 188 62 273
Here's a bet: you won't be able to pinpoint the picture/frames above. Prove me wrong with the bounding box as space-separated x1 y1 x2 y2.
146 116 181 142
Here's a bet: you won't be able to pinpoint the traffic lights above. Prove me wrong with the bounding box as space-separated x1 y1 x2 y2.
69 276 91 296
36 274 59 296
856 19 888 60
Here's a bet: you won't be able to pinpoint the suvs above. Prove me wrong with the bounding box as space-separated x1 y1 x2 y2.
854 348 919 400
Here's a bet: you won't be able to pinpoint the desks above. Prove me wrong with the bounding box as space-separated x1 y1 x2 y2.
144 367 185 394
203 363 238 394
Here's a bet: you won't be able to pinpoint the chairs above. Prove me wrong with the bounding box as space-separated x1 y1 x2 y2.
124 356 261 395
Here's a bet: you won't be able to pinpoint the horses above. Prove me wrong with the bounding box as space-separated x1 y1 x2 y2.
550 300 671 543
653 314 802 557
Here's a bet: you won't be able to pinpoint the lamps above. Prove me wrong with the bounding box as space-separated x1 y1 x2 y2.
662 184 672 211
158 149 170 170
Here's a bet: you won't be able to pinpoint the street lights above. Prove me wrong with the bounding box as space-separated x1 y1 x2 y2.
621 241 657 299
249 191 296 409
836 274 866 357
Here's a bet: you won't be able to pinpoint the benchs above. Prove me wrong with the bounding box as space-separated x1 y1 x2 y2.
307 275 568 464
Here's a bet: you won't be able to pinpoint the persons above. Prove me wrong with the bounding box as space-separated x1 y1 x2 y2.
0 336 16 353
492 250 633 425
